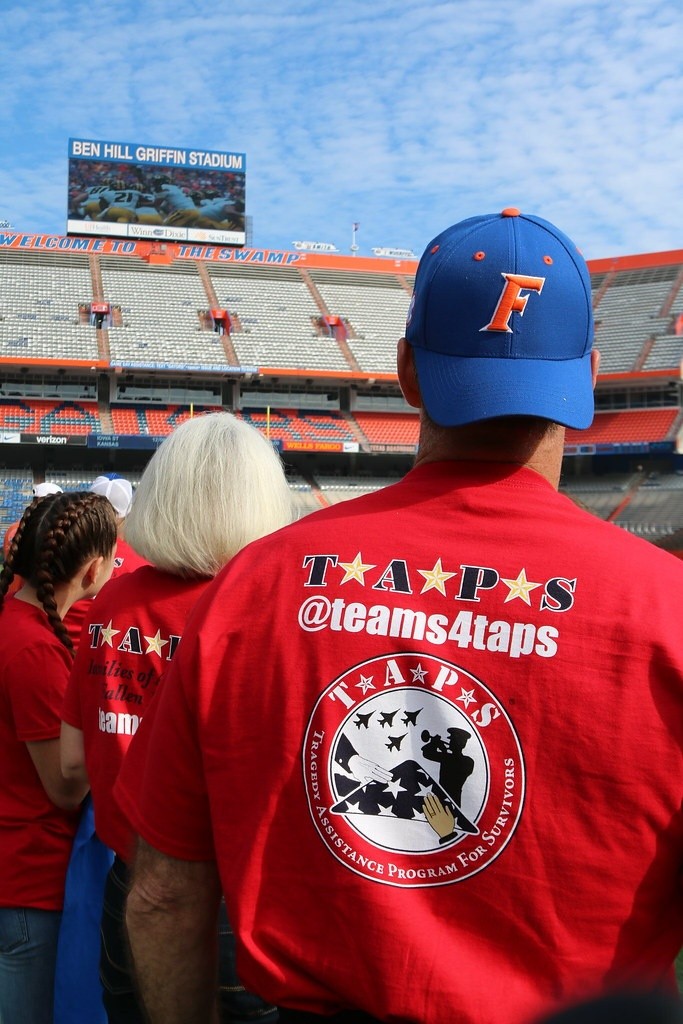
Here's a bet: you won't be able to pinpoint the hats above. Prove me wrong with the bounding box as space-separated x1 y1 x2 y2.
89 472 134 517
32 482 65 497
406 209 597 432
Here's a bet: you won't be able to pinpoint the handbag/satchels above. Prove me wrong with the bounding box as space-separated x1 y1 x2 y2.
53 802 115 1024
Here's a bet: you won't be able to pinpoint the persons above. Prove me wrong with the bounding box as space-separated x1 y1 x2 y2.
59 412 293 1023
106 207 683 1023
0 490 118 1024
